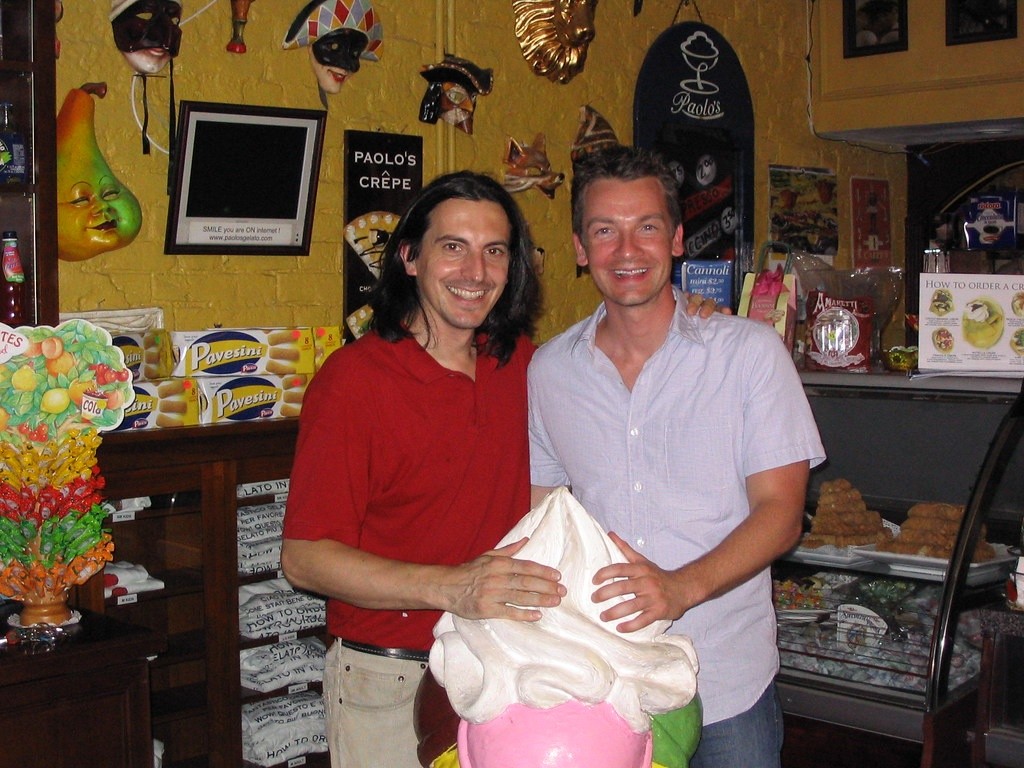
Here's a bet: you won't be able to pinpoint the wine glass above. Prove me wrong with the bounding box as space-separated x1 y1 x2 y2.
870 270 901 366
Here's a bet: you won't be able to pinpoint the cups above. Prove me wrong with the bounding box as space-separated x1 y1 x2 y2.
1007 569 1024 611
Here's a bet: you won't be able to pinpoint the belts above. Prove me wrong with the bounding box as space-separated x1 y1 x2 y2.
335 636 429 663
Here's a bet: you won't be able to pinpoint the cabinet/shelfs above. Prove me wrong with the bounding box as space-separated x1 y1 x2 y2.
0 365 1024 768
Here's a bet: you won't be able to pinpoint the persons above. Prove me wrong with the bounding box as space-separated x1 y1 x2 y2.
280 170 733 768
526 146 825 768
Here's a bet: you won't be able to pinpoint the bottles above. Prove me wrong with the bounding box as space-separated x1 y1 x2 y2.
0 101 27 185
227 0 253 52
0 232 25 324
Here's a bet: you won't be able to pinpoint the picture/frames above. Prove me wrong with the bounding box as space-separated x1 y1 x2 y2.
842 0 908 59
945 0 1017 46
164 100 328 256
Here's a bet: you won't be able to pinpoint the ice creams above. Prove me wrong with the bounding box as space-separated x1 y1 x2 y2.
426 486 703 768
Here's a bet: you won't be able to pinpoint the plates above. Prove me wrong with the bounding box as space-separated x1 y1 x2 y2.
795 551 861 566
853 544 1018 577
778 609 835 622
